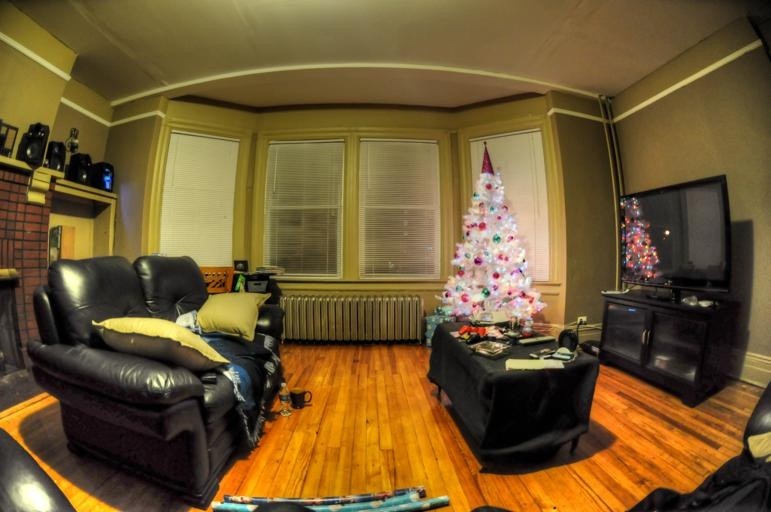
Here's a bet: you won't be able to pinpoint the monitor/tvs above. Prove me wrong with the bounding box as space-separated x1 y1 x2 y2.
620 174 732 302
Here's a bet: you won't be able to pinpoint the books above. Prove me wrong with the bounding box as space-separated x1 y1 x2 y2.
467 339 565 373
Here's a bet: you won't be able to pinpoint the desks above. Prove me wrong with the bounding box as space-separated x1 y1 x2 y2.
434 321 599 473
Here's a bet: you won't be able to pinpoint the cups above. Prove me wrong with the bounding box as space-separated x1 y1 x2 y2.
290 388 312 409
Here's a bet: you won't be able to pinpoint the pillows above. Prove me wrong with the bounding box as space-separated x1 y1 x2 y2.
90 317 232 369
195 293 274 343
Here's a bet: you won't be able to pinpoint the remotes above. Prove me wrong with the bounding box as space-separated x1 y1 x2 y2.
201 372 216 382
519 335 556 346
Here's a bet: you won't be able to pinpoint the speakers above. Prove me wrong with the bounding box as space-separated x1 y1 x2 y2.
43 141 66 172
66 153 92 186
22 122 50 166
92 161 114 192
559 329 577 352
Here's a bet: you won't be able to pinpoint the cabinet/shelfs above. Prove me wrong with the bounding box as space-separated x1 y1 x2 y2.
598 289 742 408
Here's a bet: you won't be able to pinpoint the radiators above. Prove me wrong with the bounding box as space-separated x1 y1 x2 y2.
280 295 424 344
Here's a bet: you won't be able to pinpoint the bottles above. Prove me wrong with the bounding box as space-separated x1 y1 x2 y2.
278 383 292 416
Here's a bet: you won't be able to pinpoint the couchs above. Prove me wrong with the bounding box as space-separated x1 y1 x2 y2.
27 254 287 512
